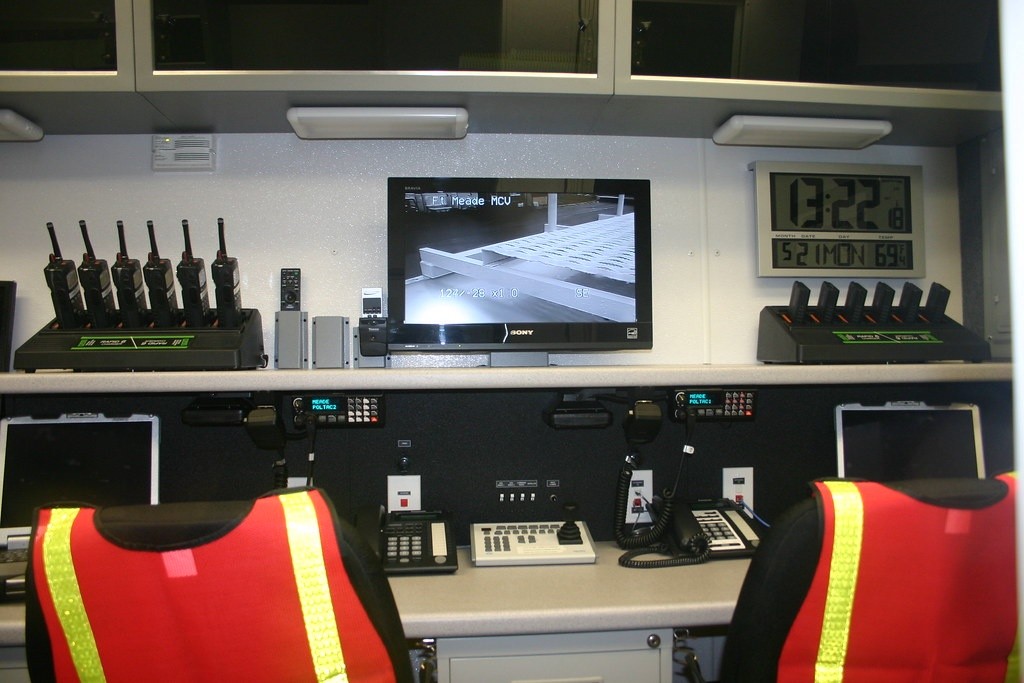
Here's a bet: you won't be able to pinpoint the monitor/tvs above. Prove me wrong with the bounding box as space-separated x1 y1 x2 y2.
387 177 653 350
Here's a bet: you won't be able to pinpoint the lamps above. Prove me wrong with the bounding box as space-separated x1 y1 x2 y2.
286 107 474 142
0 111 45 141
713 116 893 150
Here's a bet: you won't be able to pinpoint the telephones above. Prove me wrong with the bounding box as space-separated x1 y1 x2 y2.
645 495 762 559
366 501 458 576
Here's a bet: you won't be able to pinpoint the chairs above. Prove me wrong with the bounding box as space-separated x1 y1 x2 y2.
719 464 1021 682
25 486 420 683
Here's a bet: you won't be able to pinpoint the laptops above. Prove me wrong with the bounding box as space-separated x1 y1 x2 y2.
836 401 986 481
0 411 159 579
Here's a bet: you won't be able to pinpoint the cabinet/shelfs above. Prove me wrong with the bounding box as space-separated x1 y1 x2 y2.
435 631 677 683
